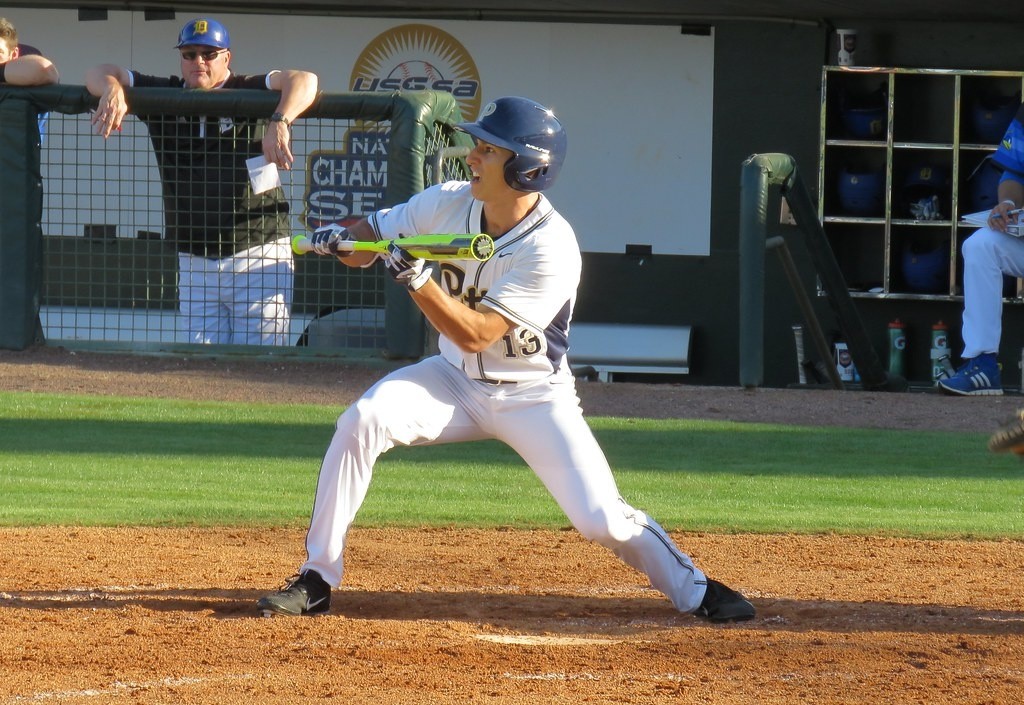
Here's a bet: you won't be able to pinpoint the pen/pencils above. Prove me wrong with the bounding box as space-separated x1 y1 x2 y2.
991 208 1023 218
90 108 123 131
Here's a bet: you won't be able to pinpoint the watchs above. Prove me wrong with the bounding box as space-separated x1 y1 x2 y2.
269 112 290 127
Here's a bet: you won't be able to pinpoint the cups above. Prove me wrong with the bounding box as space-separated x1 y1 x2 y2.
836 28 858 66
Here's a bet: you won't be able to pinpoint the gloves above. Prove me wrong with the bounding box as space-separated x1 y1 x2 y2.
384 234 434 291
311 223 357 256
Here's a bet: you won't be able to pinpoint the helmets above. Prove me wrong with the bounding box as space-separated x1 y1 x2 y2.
453 96 567 193
839 85 1020 293
175 17 231 49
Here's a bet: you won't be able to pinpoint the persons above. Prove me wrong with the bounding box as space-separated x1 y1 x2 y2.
0 17 61 144
255 95 757 625
84 18 319 349
939 100 1024 397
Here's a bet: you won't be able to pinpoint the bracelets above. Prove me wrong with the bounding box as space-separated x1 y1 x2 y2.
998 199 1016 208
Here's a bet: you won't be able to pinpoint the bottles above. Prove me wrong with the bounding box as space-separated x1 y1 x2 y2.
887 319 907 376
832 337 854 382
931 319 951 384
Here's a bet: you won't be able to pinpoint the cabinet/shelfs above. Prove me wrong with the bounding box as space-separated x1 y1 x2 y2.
814 65 1024 304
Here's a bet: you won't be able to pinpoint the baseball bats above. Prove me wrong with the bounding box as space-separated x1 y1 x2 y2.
291 233 495 263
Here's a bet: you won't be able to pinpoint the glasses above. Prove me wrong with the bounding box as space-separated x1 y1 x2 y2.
179 48 227 60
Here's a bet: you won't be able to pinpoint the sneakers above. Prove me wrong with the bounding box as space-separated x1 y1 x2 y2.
938 354 1004 397
693 579 756 624
257 568 331 616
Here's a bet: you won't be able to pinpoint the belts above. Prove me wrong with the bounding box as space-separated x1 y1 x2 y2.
477 378 517 386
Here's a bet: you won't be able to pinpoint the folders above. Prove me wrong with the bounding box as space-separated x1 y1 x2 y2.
961 207 1024 237
